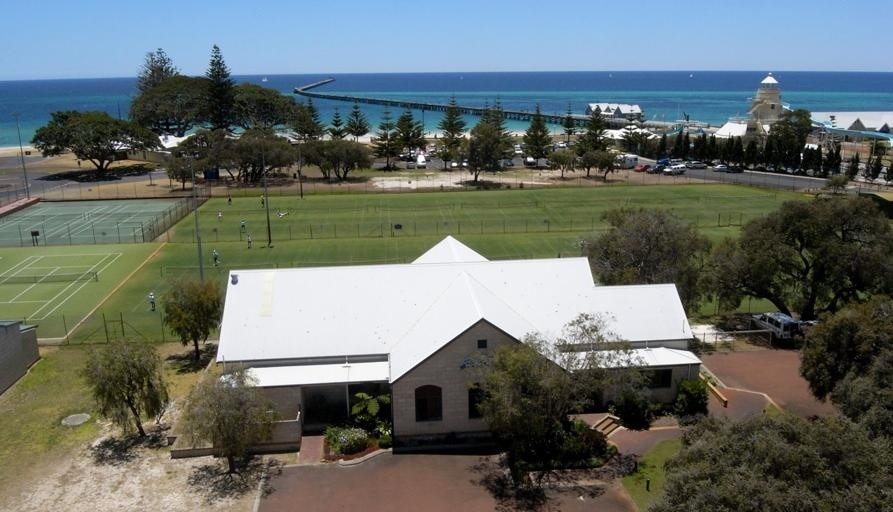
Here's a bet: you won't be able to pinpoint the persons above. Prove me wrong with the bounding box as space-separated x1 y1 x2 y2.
240 217 247 231
247 234 252 248
226 194 232 207
260 195 265 208
148 292 156 312
216 211 224 222
212 249 221 266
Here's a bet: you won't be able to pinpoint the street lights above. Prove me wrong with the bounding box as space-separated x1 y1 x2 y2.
12 112 30 199
255 134 271 247
295 135 303 198
175 151 203 282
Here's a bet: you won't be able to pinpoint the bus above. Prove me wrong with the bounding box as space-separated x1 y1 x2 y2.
614 154 639 169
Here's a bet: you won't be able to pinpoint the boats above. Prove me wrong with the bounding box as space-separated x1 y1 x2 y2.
262 76 269 82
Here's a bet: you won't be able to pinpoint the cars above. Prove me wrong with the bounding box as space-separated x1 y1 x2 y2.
515 142 537 166
634 157 708 175
713 164 727 172
556 140 580 148
450 158 458 168
504 159 514 166
462 159 469 167
398 142 443 169
753 312 820 344
606 147 619 153
